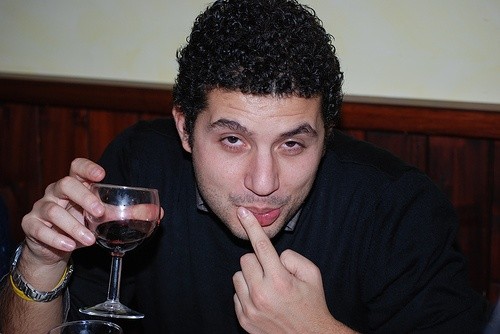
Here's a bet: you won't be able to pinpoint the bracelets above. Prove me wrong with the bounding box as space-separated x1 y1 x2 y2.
11 268 67 300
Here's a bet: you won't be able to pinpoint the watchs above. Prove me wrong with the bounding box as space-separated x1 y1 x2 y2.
9 238 74 303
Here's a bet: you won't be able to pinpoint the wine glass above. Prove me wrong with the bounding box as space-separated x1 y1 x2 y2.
79 182 161 319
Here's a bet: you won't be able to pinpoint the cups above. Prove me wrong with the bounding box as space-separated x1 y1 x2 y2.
48 320 123 334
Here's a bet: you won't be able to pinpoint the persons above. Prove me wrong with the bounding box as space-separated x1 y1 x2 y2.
0 0 490 334
